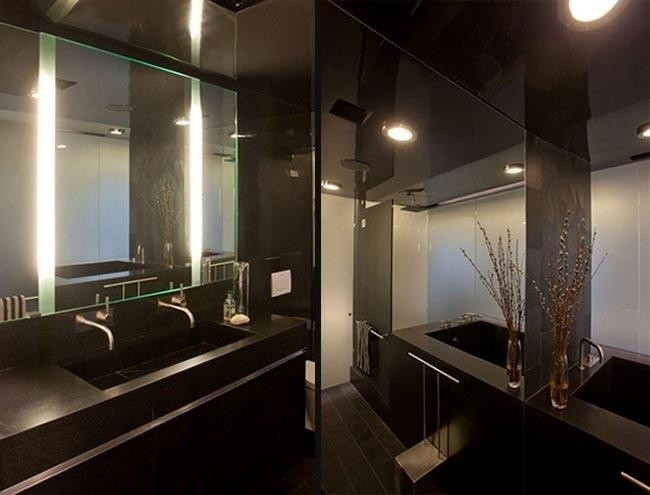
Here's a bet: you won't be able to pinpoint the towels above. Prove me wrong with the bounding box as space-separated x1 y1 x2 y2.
357 320 378 378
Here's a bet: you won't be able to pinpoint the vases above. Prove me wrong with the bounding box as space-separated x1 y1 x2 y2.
163 242 176 267
551 344 571 409
508 337 521 391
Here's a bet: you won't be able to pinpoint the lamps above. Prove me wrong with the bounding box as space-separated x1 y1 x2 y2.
503 163 524 174
110 128 124 136
173 114 191 127
634 121 650 140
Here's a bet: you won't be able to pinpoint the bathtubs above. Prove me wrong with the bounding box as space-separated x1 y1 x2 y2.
56 260 151 279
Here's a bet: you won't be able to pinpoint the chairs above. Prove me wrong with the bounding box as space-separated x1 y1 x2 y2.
391 353 461 495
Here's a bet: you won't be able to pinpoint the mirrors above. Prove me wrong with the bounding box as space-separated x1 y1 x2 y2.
0 15 237 326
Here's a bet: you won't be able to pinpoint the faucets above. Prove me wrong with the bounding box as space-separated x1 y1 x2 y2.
137 244 145 264
76 315 115 351
457 312 475 322
158 299 195 330
577 337 604 370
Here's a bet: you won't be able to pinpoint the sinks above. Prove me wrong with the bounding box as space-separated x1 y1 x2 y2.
425 320 525 378
58 320 258 391
569 355 650 428
203 251 222 257
